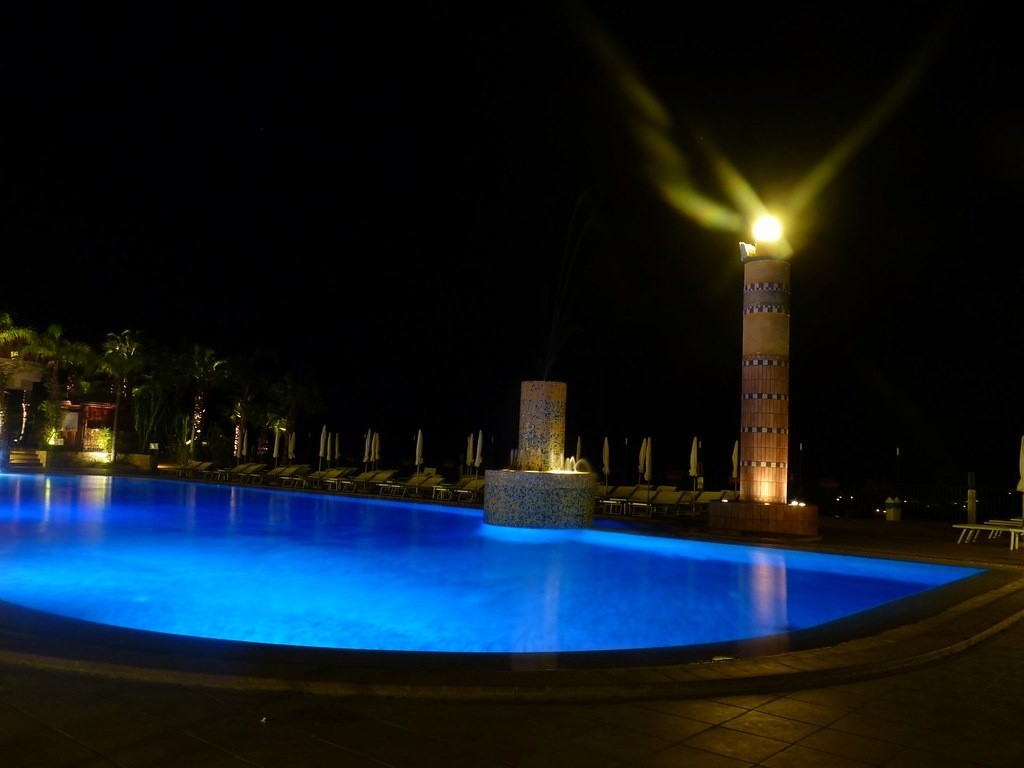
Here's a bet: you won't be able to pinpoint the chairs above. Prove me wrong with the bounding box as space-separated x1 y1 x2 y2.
167 461 485 504
952 517 1024 550
595 482 740 520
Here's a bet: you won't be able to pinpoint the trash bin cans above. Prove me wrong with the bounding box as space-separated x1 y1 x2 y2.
885 497 902 522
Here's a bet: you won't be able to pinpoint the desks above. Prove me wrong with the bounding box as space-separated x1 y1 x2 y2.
330 478 348 491
217 468 230 480
